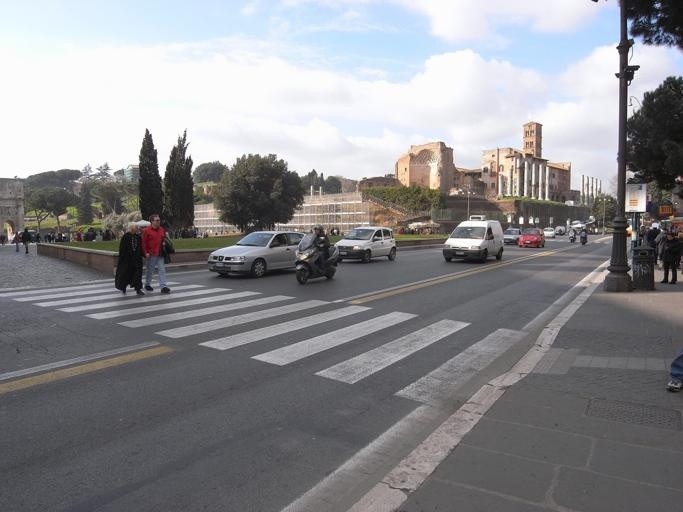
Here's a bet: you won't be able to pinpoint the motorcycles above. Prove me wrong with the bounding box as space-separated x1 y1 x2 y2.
293 231 339 284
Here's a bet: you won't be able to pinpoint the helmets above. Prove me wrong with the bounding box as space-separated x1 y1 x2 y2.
314 224 323 230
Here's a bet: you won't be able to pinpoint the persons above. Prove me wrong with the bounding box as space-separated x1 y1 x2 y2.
139 214 171 293
113 222 146 296
663 349 683 395
578 227 588 243
309 226 346 237
568 226 576 240
635 224 683 284
398 224 440 236
0 223 239 255
312 224 331 273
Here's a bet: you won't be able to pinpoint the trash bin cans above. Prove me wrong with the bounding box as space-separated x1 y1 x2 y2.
632 247 654 290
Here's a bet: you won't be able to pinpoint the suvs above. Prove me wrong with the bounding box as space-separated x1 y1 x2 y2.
332 226 396 264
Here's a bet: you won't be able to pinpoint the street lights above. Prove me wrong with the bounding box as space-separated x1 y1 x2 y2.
461 188 475 218
597 197 612 238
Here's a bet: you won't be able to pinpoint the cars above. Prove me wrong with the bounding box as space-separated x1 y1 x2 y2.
206 230 307 281
0 228 70 246
499 223 589 249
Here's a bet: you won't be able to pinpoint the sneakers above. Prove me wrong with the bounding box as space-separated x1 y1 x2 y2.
670 280 677 284
661 279 668 283
15 250 19 251
666 378 682 392
161 287 170 293
26 252 28 253
319 269 325 272
136 290 145 295
145 286 153 290
123 290 126 295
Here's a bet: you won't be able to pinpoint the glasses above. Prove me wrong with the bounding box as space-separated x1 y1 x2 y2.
153 219 160 222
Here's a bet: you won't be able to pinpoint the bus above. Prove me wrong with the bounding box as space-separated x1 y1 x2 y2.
625 217 634 235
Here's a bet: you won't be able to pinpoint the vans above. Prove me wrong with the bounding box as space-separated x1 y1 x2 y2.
439 218 504 263
